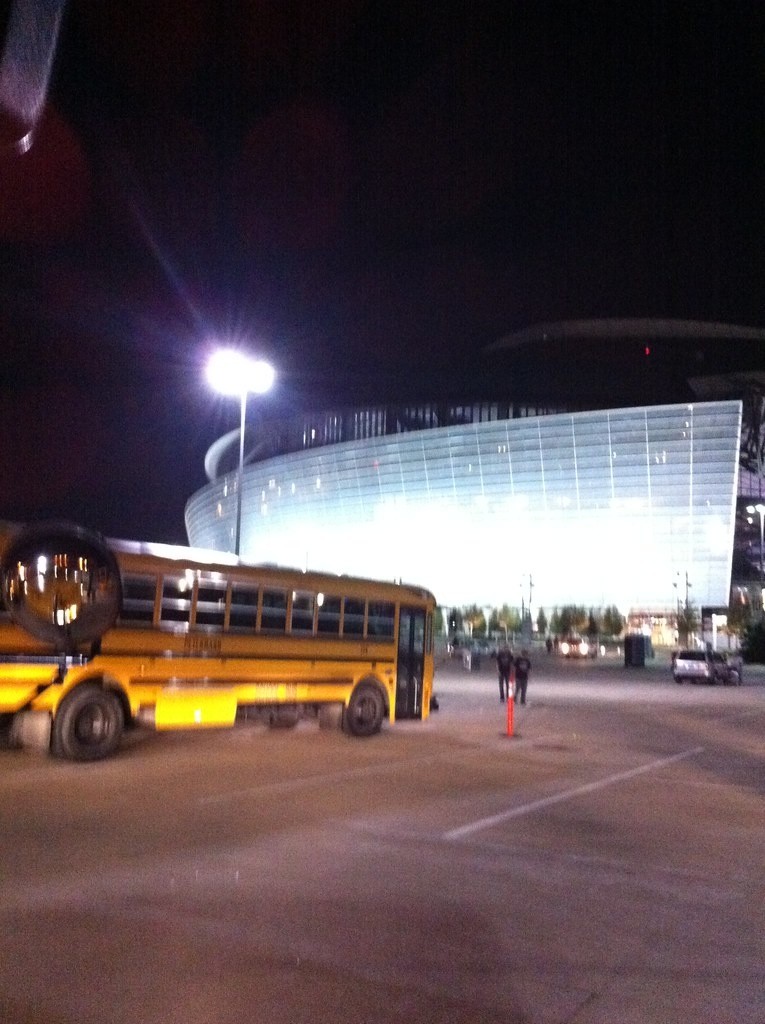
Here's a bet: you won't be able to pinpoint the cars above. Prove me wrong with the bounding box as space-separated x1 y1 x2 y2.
563 637 597 659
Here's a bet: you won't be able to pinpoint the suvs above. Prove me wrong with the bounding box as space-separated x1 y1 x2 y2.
674 649 732 685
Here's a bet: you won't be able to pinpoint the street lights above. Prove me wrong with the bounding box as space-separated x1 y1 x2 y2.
207 350 276 552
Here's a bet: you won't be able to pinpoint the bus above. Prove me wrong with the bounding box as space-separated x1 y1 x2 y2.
0 526 459 763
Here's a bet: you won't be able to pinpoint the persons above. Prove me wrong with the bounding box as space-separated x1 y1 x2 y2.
545 636 552 654
554 635 558 649
513 649 531 705
567 635 577 653
84 570 98 604
496 646 515 702
